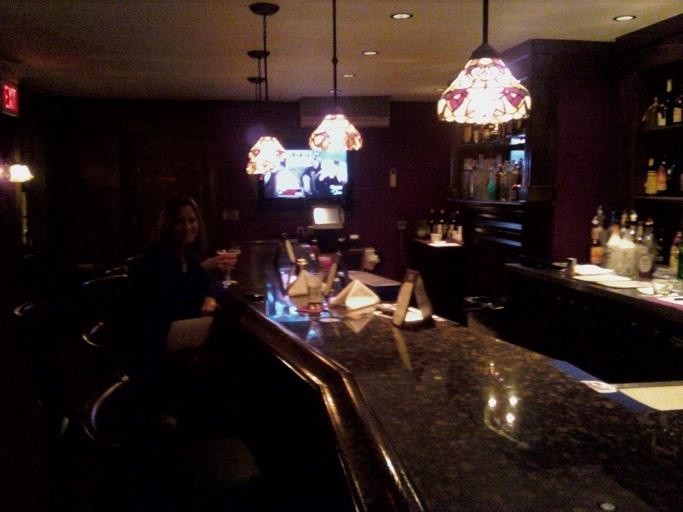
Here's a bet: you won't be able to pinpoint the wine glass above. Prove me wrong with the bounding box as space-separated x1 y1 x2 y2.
216 248 242 288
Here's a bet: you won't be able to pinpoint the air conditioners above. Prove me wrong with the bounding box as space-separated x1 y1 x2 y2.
300 96 391 128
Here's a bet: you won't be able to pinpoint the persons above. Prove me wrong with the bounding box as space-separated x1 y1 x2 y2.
299 158 322 197
111 191 242 437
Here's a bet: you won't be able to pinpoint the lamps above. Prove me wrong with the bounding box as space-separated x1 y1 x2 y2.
436 1 534 127
308 60 365 152
245 1 288 176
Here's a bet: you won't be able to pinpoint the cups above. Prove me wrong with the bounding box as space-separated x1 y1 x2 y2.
307 274 323 310
654 280 672 295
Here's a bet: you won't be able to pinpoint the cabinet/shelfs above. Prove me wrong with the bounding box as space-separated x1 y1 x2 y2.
634 124 683 201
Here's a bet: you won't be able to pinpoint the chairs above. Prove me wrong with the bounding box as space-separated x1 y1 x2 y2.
12 257 263 512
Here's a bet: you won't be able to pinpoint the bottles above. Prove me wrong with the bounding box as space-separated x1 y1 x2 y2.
461 120 524 201
590 204 683 278
426 209 462 240
642 77 683 196
565 258 578 276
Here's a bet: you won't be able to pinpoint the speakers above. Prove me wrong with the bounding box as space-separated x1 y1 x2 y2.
258 148 354 212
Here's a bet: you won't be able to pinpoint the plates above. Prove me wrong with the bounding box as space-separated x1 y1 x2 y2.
637 288 653 295
552 262 651 287
